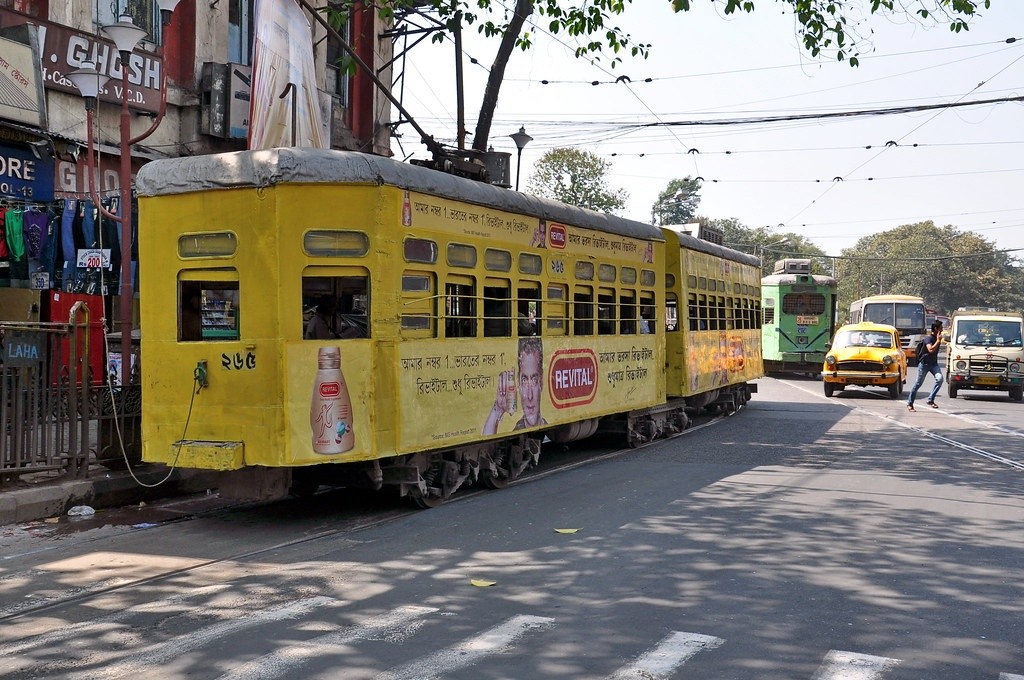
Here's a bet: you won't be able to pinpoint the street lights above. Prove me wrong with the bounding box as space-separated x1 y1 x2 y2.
760 237 790 277
509 124 534 192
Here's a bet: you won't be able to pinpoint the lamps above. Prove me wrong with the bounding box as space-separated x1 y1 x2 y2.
27 139 81 164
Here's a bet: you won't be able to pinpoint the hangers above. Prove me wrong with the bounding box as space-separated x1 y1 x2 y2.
0 188 124 214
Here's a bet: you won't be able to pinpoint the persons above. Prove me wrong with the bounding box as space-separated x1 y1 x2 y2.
305 294 343 338
673 307 705 330
1000 327 1010 340
907 321 943 412
789 295 813 315
519 312 533 336
850 333 866 344
484 338 547 437
529 219 547 249
640 306 650 334
958 325 978 343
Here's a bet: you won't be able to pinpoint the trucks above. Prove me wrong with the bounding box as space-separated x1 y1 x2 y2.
943 310 1024 399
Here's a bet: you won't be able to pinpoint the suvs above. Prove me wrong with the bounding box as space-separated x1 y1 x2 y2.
820 321 908 396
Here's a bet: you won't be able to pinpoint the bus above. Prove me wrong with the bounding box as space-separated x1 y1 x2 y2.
926 314 951 349
759 258 838 377
848 294 937 369
128 145 766 518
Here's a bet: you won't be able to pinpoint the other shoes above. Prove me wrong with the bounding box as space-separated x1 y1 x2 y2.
907 404 916 412
926 401 939 408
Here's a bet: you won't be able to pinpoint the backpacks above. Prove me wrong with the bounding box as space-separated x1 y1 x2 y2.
916 340 923 362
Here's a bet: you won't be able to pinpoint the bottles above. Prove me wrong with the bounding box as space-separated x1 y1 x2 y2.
506 372 517 413
310 346 355 454
402 190 411 226
